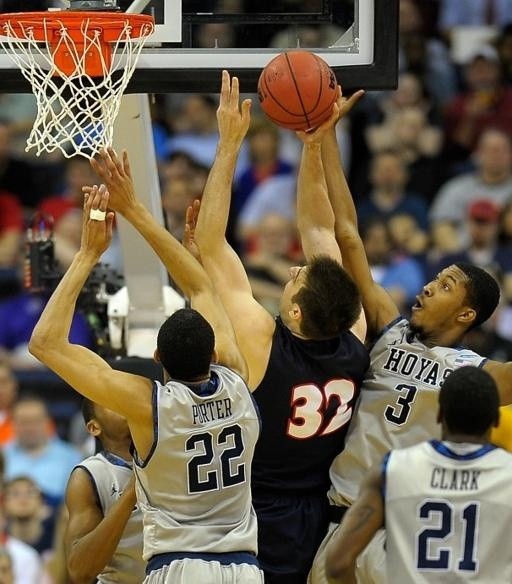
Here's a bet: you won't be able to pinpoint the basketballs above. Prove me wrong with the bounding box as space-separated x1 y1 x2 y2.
257 50 338 130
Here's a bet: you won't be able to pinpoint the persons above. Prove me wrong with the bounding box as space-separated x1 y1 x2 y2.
192 67 369 584
324 366 511 584
304 85 511 584
64 396 148 583
0 91 130 584
29 147 272 584
146 0 512 362
